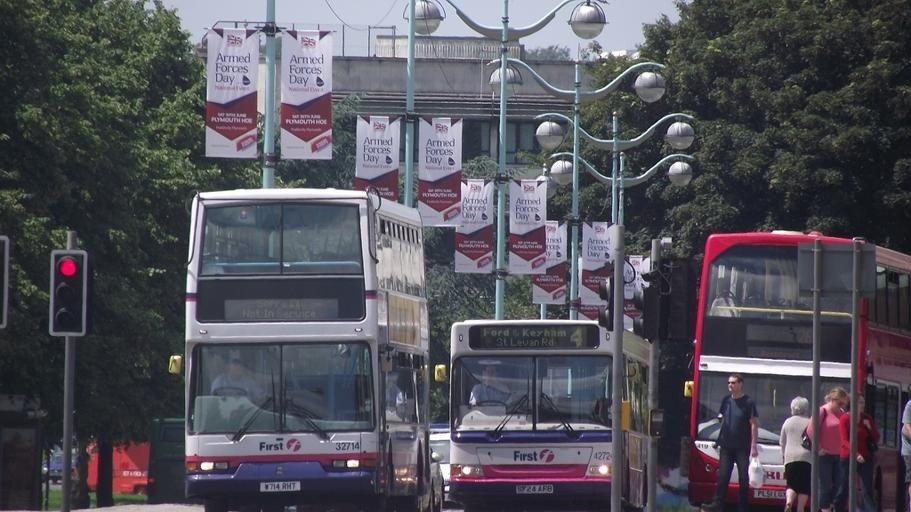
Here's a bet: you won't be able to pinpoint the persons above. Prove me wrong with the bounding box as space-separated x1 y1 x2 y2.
833 391 880 512
211 357 261 405
778 394 812 511
701 373 760 510
805 387 851 512
5 440 34 511
899 399 911 510
466 364 512 410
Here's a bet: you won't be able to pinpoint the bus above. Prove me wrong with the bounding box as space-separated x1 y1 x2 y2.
684 229 911 512
41 418 185 496
168 187 431 512
434 319 650 512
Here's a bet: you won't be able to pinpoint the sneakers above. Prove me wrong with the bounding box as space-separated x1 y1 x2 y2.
702 502 719 512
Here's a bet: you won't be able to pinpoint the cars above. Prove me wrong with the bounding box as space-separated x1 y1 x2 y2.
429 424 456 512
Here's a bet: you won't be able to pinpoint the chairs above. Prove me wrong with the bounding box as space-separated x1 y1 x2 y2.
710 291 741 318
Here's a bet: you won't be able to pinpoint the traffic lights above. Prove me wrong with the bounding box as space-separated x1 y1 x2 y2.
48 249 88 337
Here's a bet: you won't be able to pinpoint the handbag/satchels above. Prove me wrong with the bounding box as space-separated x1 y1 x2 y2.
866 436 878 452
802 427 812 450
